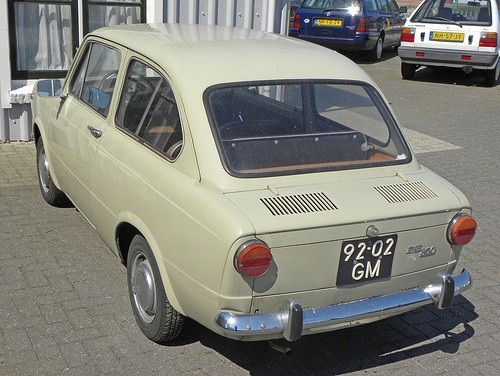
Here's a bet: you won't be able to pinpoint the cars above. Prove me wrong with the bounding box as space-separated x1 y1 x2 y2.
26 22 478 349
289 0 410 62
397 0 500 86
